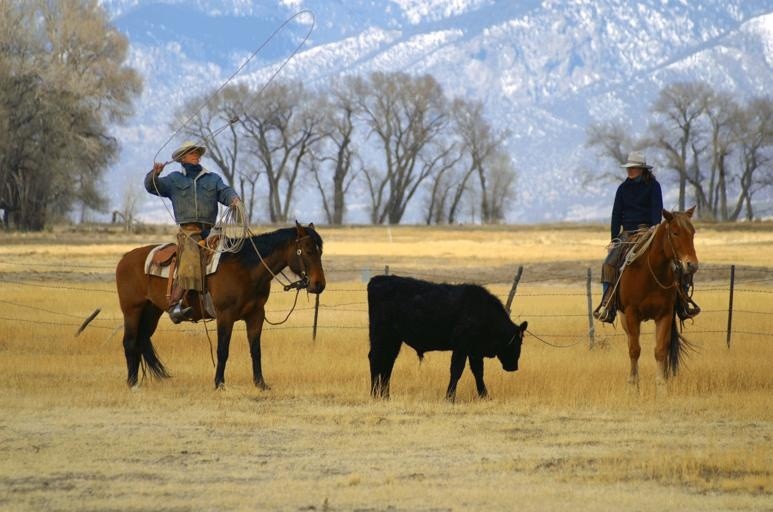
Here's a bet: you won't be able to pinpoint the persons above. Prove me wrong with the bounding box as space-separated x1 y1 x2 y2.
144 141 243 325
589 151 700 324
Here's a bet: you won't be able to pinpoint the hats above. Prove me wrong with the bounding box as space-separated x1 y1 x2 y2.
618 152 654 170
172 141 207 162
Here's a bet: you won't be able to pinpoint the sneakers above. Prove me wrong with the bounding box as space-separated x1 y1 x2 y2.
679 306 700 321
170 306 193 324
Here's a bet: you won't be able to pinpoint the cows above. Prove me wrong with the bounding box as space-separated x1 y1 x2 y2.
365 275 528 403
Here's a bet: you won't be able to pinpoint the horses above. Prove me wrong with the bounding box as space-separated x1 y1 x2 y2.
615 204 705 397
115 219 326 390
